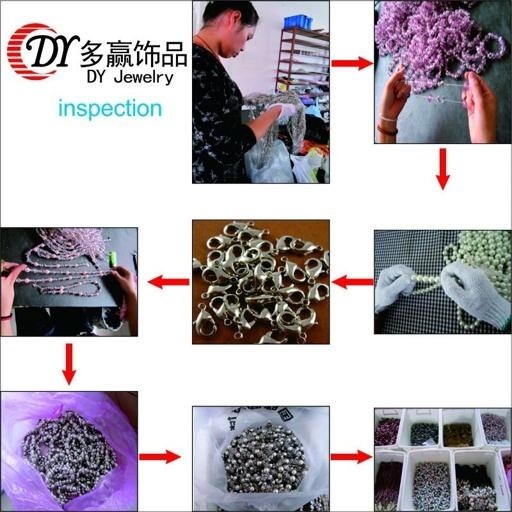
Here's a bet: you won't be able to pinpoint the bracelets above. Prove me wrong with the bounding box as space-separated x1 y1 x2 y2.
0 313 16 322
379 126 400 137
377 111 400 125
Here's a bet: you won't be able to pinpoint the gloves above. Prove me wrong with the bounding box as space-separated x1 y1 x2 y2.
375 264 418 315
441 262 512 331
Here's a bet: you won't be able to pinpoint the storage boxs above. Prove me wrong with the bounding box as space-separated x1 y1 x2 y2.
284 15 313 32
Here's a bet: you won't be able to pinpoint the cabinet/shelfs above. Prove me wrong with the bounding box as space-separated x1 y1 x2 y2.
275 27 331 95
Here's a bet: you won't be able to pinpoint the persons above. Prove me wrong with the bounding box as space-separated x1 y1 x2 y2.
0 260 138 337
191 1 295 184
374 260 511 335
375 64 499 143
